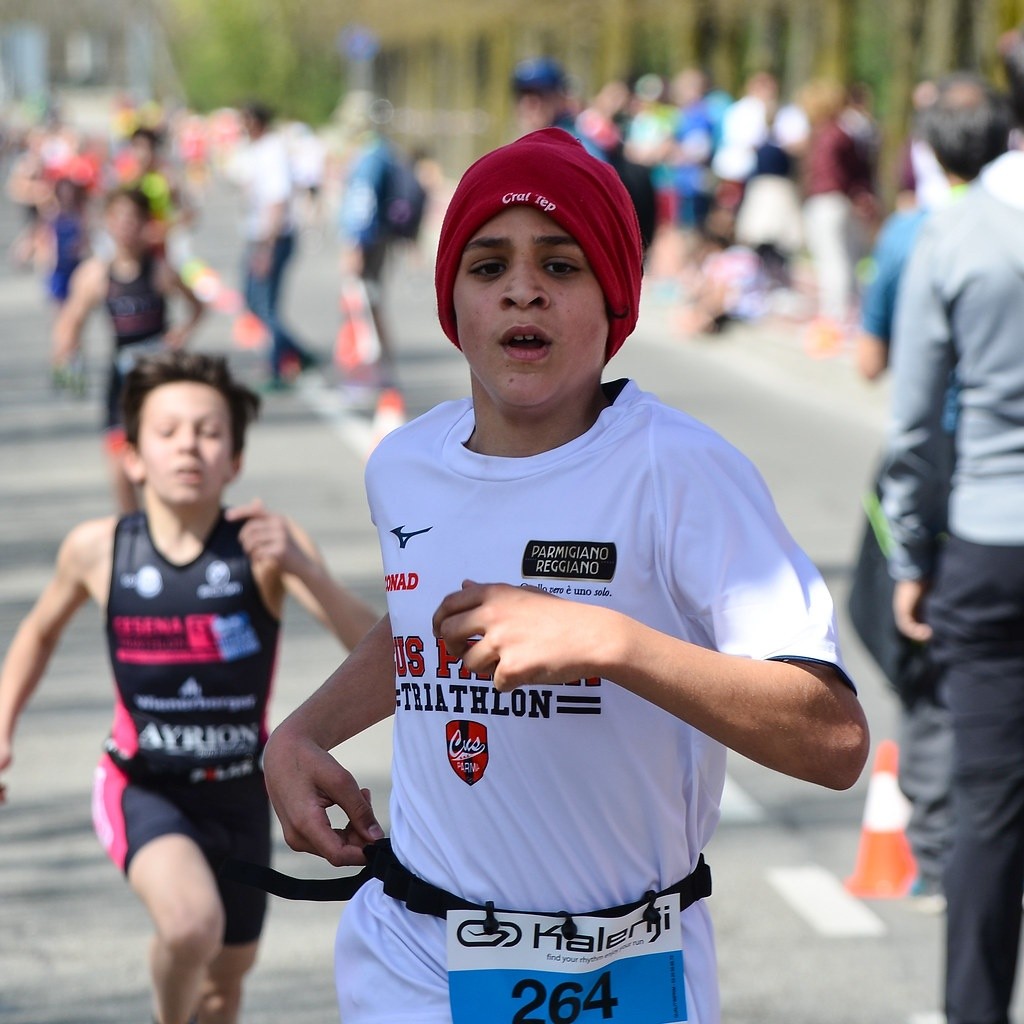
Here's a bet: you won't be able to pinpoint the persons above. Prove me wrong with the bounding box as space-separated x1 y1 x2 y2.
0 347 382 1024
261 128 870 1024
859 26 1024 1024
28 95 420 441
511 55 884 349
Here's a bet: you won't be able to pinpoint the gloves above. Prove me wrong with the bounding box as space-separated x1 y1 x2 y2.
843 454 928 693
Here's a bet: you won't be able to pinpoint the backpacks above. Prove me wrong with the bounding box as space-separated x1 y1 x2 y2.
371 163 425 241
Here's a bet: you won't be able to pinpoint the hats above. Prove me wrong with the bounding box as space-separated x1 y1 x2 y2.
433 128 643 365
509 59 562 100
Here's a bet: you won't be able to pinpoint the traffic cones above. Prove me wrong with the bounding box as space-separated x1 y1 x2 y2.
842 739 930 901
365 384 411 455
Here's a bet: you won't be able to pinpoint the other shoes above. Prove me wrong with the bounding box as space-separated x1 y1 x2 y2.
909 871 950 914
